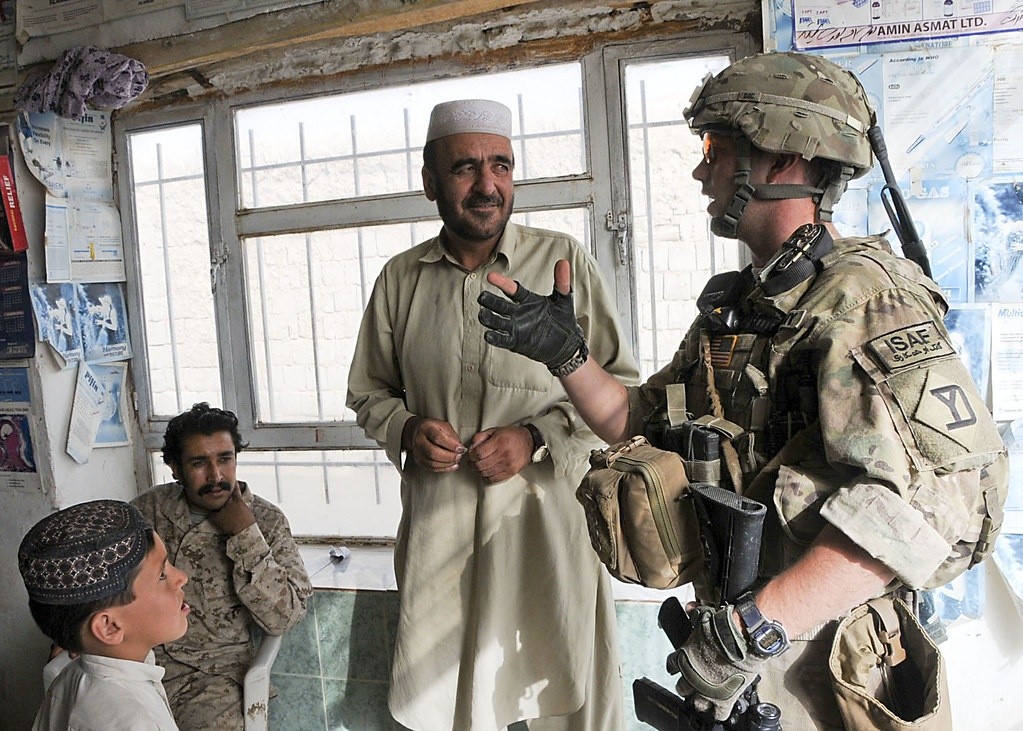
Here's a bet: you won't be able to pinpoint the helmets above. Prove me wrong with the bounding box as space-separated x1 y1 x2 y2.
683 49 876 181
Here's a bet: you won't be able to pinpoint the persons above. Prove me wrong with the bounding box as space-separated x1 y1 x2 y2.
131 402 313 731
478 52 1010 731
18 499 191 731
345 98 639 731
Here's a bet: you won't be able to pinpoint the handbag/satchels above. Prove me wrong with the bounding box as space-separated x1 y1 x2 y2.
827 599 952 731
576 436 703 590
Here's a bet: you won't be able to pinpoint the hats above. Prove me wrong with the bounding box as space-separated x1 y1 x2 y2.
17 499 155 606
425 100 513 142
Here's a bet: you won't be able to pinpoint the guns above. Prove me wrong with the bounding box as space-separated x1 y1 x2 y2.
631 480 783 731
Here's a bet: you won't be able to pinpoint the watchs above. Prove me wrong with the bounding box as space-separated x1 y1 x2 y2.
523 424 549 463
735 592 792 659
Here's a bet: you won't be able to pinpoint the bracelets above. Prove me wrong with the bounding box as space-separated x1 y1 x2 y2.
548 339 590 377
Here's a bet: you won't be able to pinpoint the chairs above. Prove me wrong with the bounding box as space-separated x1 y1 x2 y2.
41 631 283 731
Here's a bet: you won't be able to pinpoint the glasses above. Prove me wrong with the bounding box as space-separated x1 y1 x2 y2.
701 134 748 170
